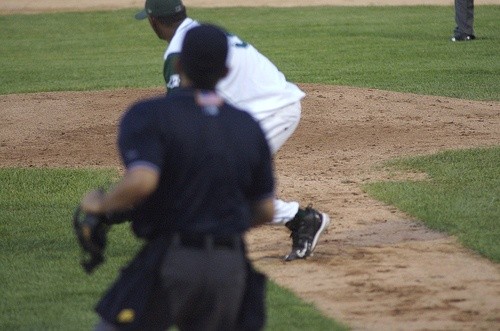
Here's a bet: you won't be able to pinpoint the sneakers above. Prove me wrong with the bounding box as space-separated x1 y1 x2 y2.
284 208 329 262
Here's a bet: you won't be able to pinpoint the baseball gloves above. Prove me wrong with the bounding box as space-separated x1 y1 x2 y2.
72 186 110 276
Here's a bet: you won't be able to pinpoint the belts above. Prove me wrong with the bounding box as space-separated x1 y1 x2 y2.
180 234 242 252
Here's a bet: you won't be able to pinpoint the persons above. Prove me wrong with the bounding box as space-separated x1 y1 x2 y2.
81 25 274 331
451 0 475 42
135 0 330 263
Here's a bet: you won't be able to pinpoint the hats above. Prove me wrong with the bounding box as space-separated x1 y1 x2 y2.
181 25 229 67
135 0 183 20
